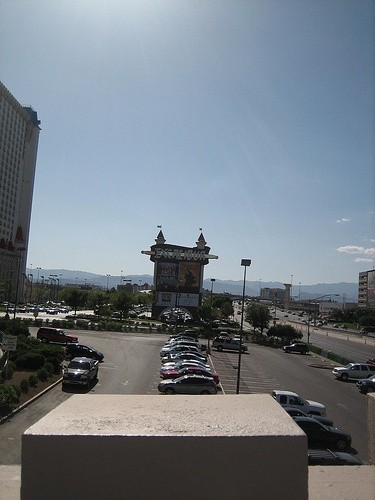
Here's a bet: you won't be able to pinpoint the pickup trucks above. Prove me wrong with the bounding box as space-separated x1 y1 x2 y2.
270 389 327 417
62 356 99 388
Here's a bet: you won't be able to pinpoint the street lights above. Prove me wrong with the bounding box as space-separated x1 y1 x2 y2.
28 274 60 303
236 258 251 394
210 278 216 328
307 293 339 342
13 247 26 320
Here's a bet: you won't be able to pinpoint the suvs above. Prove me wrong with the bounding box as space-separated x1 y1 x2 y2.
355 374 375 392
332 363 375 381
283 343 310 354
307 447 363 465
36 326 79 344
211 335 248 353
47 307 69 315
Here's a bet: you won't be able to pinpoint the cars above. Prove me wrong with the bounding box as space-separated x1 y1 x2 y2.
284 407 333 427
232 335 244 344
218 331 231 337
281 309 348 329
360 328 368 334
293 417 352 450
157 330 220 395
65 342 104 362
